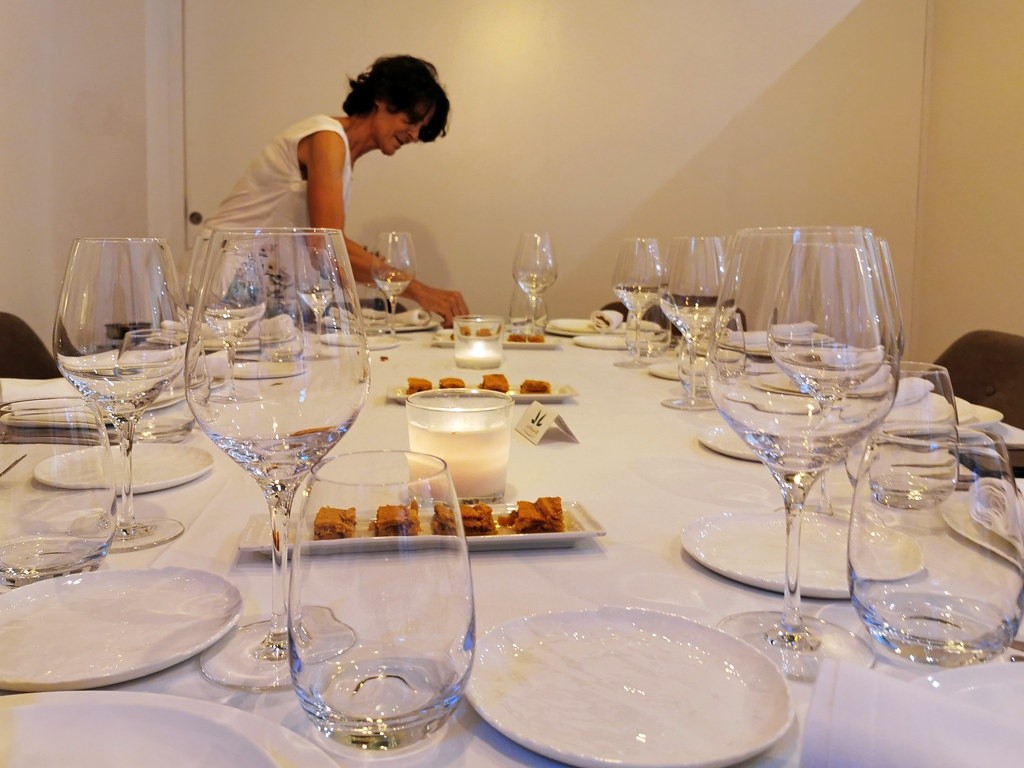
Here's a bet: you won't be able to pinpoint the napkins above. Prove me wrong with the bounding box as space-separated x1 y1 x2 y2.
159 311 296 343
194 349 234 380
591 310 623 332
772 322 817 337
721 329 769 343
890 376 935 407
330 306 429 329
2 374 163 414
791 345 887 368
968 476 1024 560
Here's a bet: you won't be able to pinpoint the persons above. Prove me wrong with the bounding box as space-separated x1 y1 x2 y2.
186 53 471 330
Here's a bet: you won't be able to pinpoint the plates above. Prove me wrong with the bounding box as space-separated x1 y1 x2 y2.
0 317 1023 768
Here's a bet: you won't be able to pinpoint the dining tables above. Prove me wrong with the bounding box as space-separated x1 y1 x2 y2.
0 317 1024 768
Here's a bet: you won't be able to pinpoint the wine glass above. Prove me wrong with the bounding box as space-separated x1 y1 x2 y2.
662 236 727 410
371 229 415 341
709 224 897 682
778 236 905 524
181 223 366 688
611 238 663 367
293 246 337 361
511 231 558 334
187 228 264 406
53 237 188 552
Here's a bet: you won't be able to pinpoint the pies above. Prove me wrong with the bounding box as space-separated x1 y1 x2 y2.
406 374 552 395
313 496 567 539
450 326 545 343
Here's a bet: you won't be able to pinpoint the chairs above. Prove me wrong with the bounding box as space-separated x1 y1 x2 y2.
934 328 1024 435
1 313 66 380
600 294 747 336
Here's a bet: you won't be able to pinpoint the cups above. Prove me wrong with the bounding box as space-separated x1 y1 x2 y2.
677 311 748 396
626 298 672 366
846 426 1022 670
0 397 120 589
119 329 210 445
452 314 505 369
845 358 960 506
356 282 388 337
283 448 477 750
507 286 547 334
405 386 516 510
259 298 302 360
